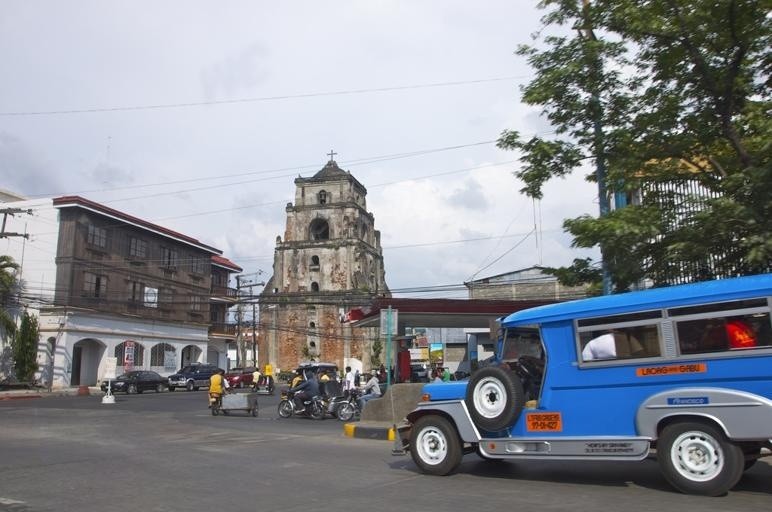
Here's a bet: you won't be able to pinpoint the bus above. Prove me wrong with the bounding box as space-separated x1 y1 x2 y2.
392 275 768 497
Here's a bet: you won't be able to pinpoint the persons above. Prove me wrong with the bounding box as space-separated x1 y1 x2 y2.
429 369 443 384
251 367 262 391
220 370 230 394
582 318 646 363
439 368 450 382
703 305 758 352
288 367 383 414
208 368 225 408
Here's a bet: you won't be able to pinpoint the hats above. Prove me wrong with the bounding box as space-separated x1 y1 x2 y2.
368 369 378 377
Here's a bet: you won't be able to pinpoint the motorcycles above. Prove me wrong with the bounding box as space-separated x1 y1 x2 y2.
277 389 361 422
209 384 272 417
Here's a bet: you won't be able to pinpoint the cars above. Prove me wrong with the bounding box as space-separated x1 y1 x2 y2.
291 363 338 382
101 362 261 394
410 362 427 381
